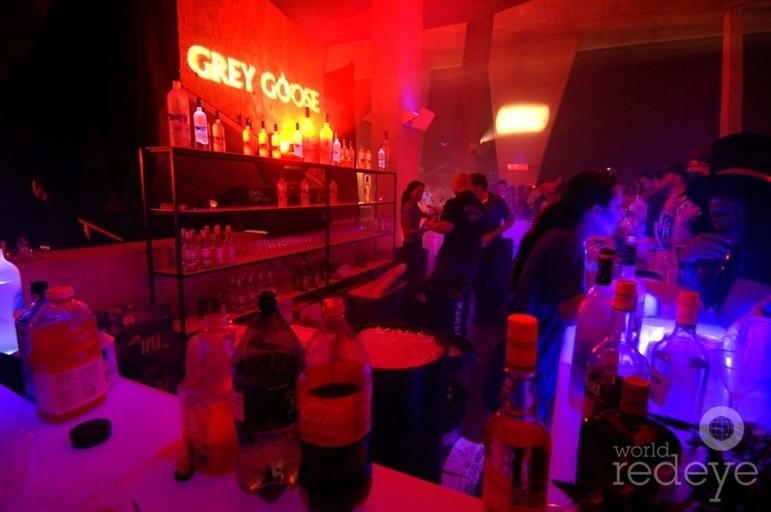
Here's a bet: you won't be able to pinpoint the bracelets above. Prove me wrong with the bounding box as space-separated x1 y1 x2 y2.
675 244 684 256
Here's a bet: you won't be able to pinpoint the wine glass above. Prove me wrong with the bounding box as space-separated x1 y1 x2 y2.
704 348 767 409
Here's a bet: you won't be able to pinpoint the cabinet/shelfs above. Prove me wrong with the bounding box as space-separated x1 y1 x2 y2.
139 143 325 381
326 165 395 296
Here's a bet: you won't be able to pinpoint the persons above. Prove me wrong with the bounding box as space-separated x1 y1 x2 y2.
422 173 484 332
677 130 771 307
471 173 516 325
399 180 432 307
628 155 702 250
490 177 558 224
482 171 620 423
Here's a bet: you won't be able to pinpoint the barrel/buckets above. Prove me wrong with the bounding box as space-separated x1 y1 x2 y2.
25 282 113 429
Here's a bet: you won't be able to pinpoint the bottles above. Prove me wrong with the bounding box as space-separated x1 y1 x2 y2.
14 279 50 406
650 287 710 427
194 97 210 151
293 123 304 157
167 73 192 147
582 279 649 418
348 141 354 162
358 146 365 168
179 224 236 270
197 270 276 314
574 377 682 511
366 148 372 170
320 111 334 163
342 139 350 162
232 290 304 500
613 242 645 349
303 107 316 160
180 314 237 475
258 121 270 157
0 247 24 354
378 146 386 168
243 118 254 156
272 123 281 158
334 131 342 163
482 313 553 510
212 109 226 152
568 248 621 402
297 298 374 509
329 178 338 206
22 280 120 489
277 173 288 207
301 177 310 206
363 176 373 204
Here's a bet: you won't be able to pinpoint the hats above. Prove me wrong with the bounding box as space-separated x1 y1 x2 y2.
686 133 771 210
